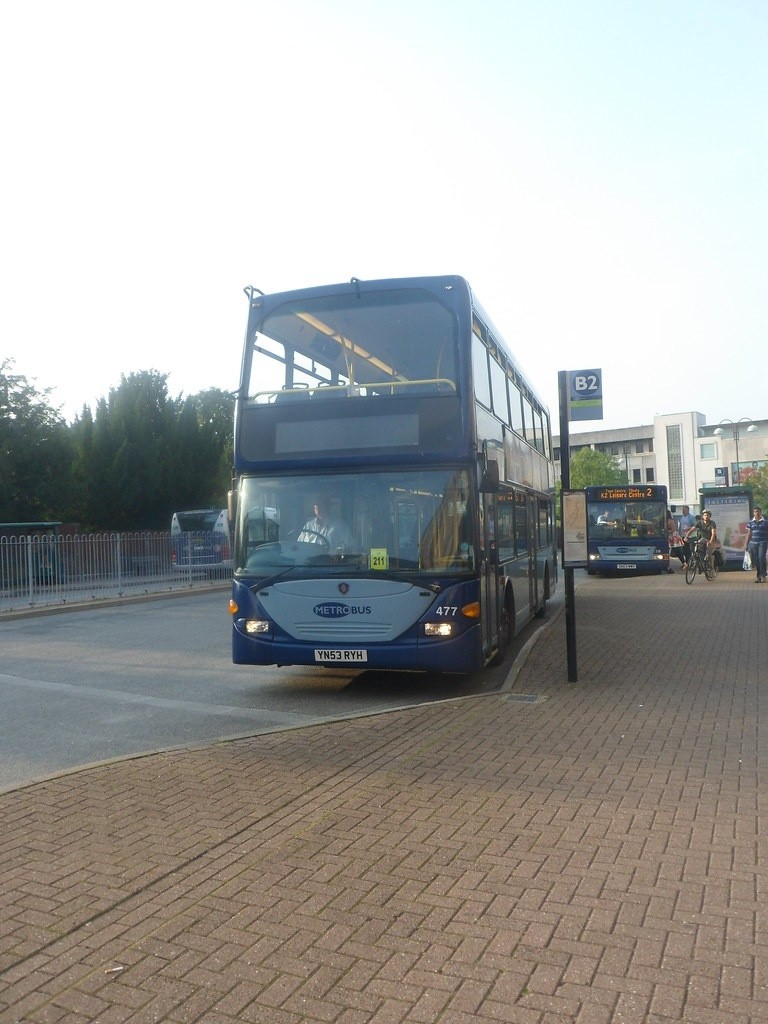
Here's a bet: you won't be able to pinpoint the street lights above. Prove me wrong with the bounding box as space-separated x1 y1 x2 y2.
714 417 760 486
617 452 628 483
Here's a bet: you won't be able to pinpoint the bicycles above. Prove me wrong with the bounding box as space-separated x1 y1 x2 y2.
682 539 718 584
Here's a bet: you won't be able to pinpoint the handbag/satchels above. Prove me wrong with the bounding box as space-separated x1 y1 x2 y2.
742 551 754 570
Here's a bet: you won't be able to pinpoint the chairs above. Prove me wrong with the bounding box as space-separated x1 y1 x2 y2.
274 373 481 564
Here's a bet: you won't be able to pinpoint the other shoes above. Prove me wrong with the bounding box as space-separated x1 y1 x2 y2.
754 577 765 583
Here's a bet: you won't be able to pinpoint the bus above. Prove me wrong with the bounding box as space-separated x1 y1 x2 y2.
227 276 559 686
0 521 65 586
172 509 232 575
582 483 671 575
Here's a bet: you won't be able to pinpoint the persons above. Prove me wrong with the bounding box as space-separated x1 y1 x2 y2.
749 462 764 487
666 510 688 570
596 507 618 526
744 507 768 583
684 509 717 579
678 506 697 564
297 495 358 556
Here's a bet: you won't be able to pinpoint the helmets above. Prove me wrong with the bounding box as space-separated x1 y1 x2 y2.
702 509 712 516
